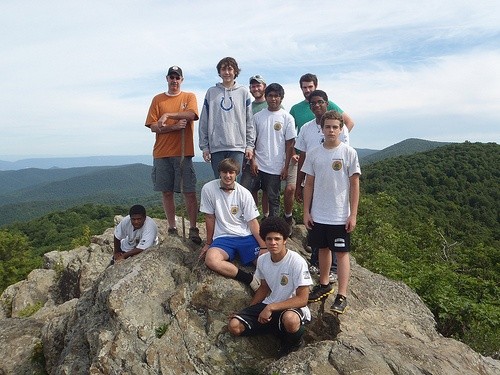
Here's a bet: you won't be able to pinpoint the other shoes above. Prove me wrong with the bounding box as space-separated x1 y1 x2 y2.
295 335 306 349
261 216 267 221
284 213 293 236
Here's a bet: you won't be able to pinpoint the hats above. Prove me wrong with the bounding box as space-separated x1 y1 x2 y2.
249 75 266 84
167 66 182 76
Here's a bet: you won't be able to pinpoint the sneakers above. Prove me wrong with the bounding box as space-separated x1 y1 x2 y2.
309 264 321 277
168 228 179 235
307 281 335 302
189 227 203 245
330 293 350 314
328 272 338 283
248 271 262 291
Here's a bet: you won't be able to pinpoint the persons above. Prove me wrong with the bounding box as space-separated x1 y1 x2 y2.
199 56 257 184
293 89 350 283
145 66 202 244
199 159 268 292
243 75 284 219
303 109 362 315
288 73 354 254
108 204 157 267
244 83 296 218
229 215 312 352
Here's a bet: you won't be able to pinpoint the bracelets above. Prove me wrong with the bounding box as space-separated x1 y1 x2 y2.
259 248 267 249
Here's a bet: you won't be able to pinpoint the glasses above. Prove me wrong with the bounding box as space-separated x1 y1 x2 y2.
218 176 235 190
309 100 327 107
169 75 182 80
129 217 144 223
267 93 282 99
